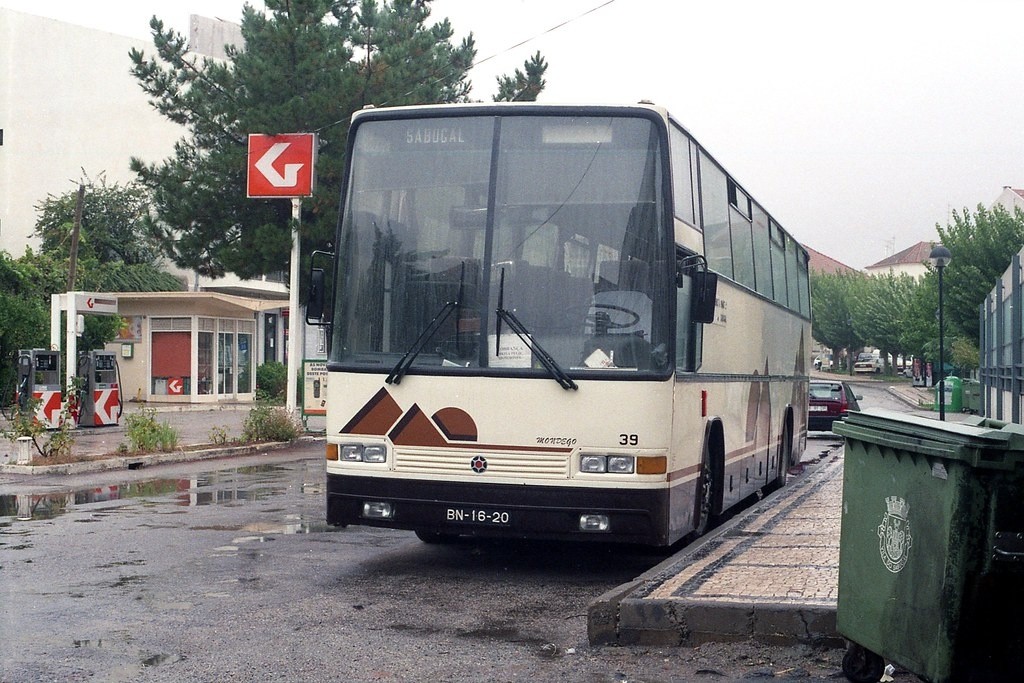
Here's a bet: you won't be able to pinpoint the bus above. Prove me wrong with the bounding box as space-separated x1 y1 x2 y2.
303 97 816 551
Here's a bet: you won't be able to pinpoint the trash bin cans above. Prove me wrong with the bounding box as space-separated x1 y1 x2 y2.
935 376 962 413
961 379 981 414
833 407 1024 683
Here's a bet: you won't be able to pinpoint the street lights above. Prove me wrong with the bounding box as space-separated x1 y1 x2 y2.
929 244 953 421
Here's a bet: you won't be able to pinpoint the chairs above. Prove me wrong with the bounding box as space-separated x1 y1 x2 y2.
492 258 652 314
403 279 477 359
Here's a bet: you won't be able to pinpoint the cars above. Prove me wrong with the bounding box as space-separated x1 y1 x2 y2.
812 347 914 378
808 379 864 432
